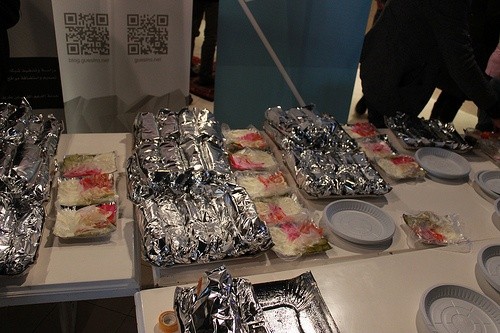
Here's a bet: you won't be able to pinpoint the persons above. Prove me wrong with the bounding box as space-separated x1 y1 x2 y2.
355 0 500 128
0 0 20 86
191 0 219 87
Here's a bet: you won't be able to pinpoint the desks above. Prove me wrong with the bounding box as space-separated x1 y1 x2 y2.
0 128 500 333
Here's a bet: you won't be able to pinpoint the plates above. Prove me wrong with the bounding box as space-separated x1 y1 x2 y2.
323 200 398 245
419 284 500 333
474 168 500 217
414 147 471 178
477 243 500 293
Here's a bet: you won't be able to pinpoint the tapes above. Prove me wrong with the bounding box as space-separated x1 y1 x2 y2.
158 310 179 332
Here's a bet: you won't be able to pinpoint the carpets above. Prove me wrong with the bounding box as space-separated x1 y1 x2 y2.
189 56 216 102
